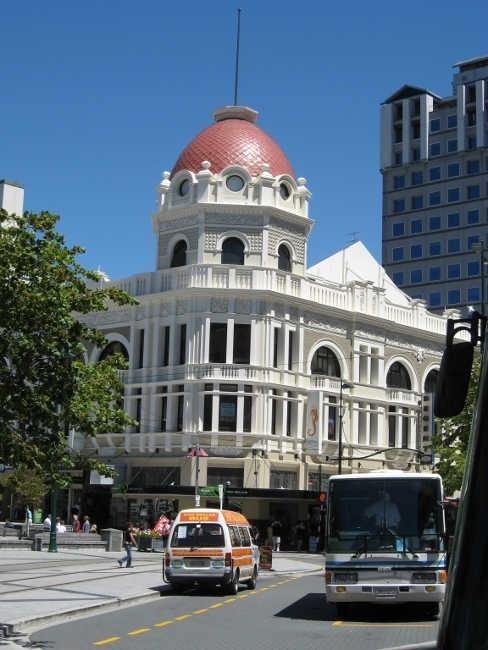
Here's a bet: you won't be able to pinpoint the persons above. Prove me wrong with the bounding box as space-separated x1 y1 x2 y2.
117 514 171 568
5 504 98 537
367 491 401 528
250 518 320 552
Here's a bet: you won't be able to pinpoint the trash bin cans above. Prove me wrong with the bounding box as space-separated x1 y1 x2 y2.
259 546 272 570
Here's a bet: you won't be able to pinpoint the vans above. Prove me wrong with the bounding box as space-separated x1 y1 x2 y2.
159 507 260 595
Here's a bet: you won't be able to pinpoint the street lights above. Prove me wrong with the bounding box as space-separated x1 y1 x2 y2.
339 377 355 474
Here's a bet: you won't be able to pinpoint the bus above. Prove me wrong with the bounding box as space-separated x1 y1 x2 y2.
323 467 449 615
